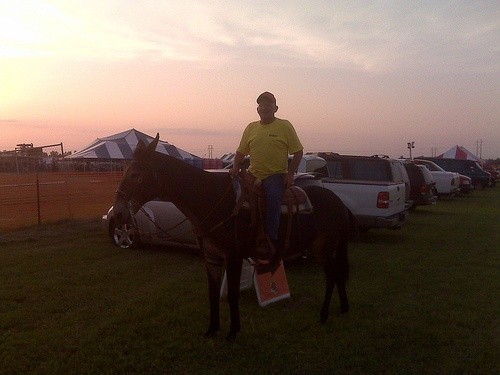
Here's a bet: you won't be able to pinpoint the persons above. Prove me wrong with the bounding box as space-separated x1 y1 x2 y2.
230 91 304 253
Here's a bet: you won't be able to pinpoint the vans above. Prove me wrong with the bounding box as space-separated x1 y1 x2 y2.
417 158 492 187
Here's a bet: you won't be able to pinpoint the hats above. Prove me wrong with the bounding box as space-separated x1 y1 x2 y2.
257 92 276 103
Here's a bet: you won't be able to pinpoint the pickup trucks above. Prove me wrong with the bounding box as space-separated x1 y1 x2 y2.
288 153 406 223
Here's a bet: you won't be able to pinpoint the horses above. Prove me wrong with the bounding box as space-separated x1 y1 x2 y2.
113 134 355 341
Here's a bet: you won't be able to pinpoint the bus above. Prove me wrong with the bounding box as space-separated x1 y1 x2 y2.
306 151 409 212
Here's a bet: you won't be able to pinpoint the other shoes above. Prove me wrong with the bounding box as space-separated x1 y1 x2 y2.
258 251 277 264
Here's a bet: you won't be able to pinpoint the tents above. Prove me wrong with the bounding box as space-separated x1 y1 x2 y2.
63 129 204 192
435 145 484 162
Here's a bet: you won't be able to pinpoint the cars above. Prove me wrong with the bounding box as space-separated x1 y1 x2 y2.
100 169 324 263
402 158 473 194
401 161 439 204
